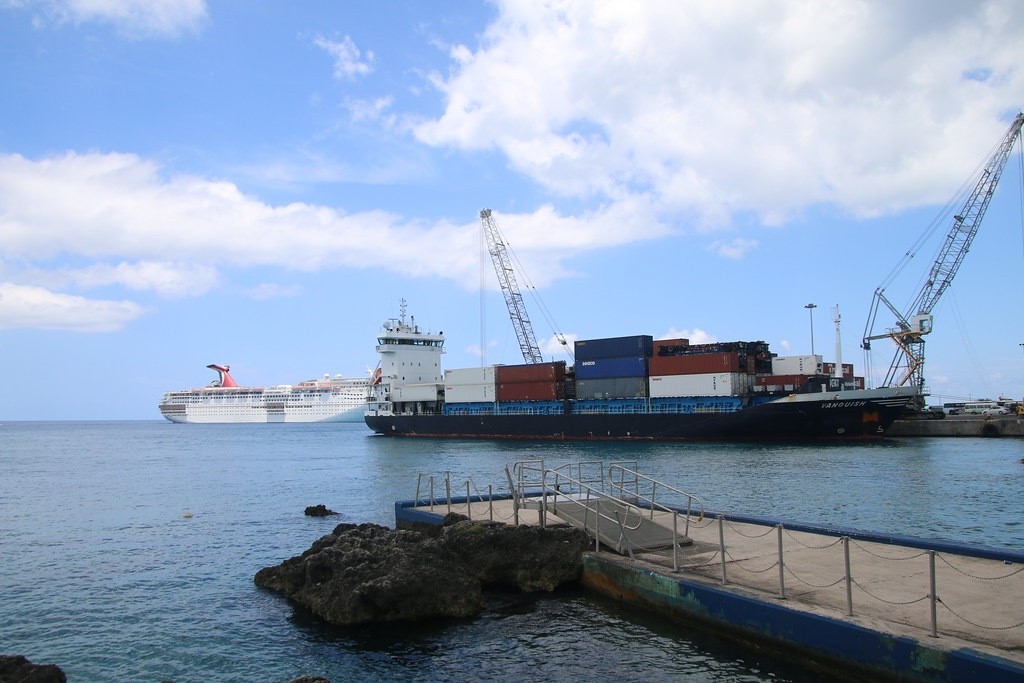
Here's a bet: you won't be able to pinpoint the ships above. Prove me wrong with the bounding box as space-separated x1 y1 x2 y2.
362 297 929 444
159 364 374 425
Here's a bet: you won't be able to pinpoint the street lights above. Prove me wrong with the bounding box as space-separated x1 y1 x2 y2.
804 303 818 355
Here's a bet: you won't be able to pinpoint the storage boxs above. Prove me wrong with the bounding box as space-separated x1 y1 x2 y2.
444 334 865 414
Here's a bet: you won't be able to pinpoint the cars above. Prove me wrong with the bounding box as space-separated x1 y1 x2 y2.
980 405 1011 416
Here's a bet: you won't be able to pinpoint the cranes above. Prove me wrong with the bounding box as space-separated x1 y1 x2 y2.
861 111 1024 418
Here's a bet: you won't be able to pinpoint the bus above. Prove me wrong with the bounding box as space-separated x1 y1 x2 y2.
964 402 998 414
997 398 1024 417
925 405 944 411
942 401 995 415
